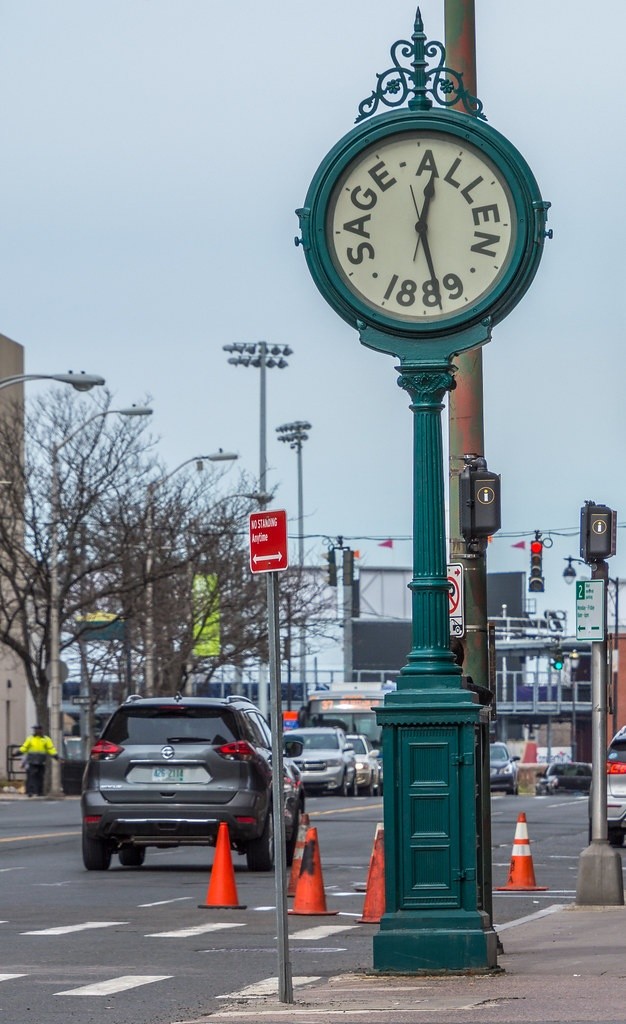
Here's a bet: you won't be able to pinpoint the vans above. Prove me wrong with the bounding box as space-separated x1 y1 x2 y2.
532 762 594 795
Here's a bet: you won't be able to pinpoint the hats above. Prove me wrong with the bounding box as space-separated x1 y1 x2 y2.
32 725 44 729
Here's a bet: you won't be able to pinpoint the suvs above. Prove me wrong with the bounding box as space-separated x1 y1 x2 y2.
281 726 358 797
79 695 306 871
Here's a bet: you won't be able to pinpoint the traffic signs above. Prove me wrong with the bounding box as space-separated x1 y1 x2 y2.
248 509 290 575
573 579 604 645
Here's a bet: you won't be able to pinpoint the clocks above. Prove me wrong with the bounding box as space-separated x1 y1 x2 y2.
294 7 553 361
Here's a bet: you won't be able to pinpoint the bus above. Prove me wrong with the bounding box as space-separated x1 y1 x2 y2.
296 679 392 779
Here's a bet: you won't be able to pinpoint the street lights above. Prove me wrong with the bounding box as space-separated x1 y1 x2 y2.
273 418 311 688
144 452 243 697
220 340 297 1003
562 556 622 741
47 406 154 799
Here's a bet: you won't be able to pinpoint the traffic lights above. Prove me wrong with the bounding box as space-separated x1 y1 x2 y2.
526 540 547 594
553 650 566 669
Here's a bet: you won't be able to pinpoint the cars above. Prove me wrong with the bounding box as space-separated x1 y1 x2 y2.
345 734 381 797
586 725 626 850
490 742 520 795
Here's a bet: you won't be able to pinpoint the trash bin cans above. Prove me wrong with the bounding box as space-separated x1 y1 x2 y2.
60 760 88 796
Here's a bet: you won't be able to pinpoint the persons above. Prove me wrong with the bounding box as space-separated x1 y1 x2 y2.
11 725 61 797
19 753 29 794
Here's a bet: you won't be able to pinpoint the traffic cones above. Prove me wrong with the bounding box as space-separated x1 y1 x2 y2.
288 825 340 914
494 812 551 891
353 822 384 891
196 822 248 910
286 813 312 898
354 828 385 924
